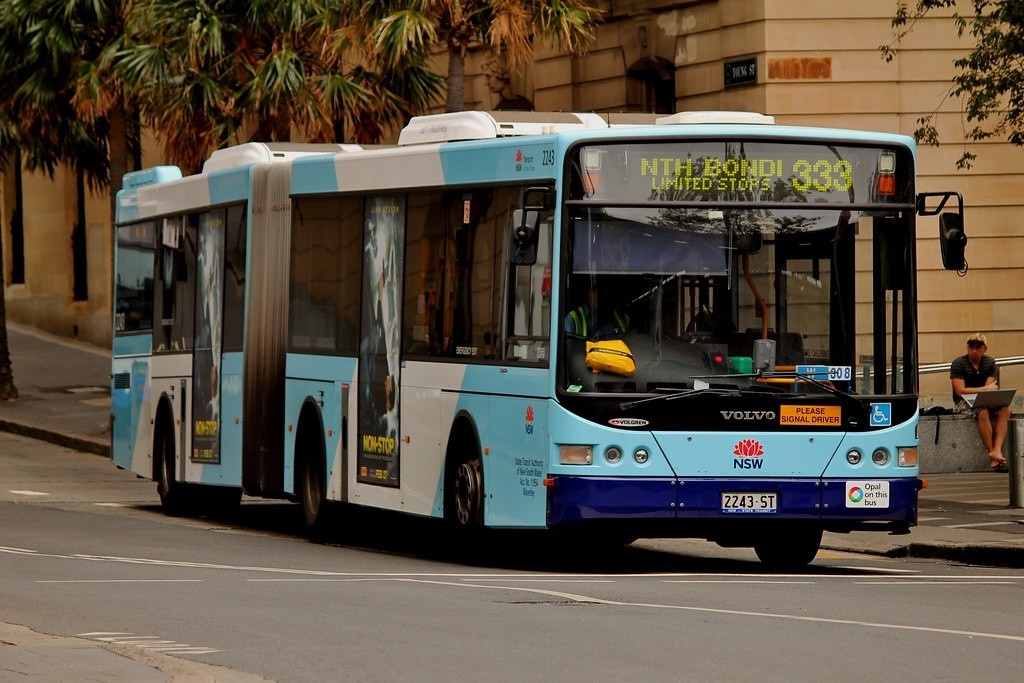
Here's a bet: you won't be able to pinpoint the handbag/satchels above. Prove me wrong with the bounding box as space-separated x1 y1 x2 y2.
919 406 961 415
585 340 635 377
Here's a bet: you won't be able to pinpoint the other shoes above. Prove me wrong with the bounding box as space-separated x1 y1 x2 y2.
993 466 1009 473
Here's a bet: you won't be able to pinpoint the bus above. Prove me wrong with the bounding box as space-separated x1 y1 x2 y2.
110 110 969 572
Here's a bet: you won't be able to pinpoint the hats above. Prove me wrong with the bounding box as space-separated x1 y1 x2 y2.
967 332 986 346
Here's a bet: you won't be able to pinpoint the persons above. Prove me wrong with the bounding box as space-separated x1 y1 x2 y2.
950 333 1011 467
564 283 636 341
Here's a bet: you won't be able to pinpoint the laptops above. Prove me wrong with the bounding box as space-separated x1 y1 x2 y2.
961 389 1017 410
